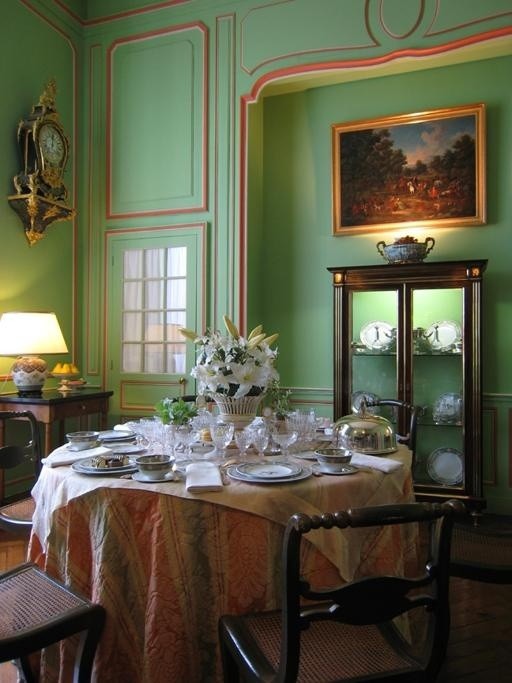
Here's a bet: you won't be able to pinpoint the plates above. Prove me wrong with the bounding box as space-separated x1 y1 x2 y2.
421 319 460 352
314 422 336 431
225 462 314 485
312 463 360 476
358 319 397 351
314 434 331 442
292 449 317 460
351 390 380 416
432 392 462 422
104 444 144 454
99 430 136 441
70 455 140 474
425 446 463 485
129 469 185 483
65 439 104 452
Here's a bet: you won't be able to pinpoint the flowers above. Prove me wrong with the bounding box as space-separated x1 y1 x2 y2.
176 315 283 400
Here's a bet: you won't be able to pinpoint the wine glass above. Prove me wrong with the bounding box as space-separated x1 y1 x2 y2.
139 407 298 465
291 408 317 443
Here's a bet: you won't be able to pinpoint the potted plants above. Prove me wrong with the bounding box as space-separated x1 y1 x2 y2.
153 397 200 433
270 378 294 434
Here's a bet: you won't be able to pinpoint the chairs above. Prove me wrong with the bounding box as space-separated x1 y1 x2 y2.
0 410 42 537
365 398 421 472
218 501 465 683
0 560 107 683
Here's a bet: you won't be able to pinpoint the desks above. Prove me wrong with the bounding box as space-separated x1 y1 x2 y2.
0 387 114 500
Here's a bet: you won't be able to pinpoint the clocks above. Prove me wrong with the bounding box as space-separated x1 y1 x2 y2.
6 72 77 247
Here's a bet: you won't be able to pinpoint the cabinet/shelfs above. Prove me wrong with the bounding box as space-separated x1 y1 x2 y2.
326 259 488 529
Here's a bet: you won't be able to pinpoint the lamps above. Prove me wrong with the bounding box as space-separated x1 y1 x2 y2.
0 311 68 397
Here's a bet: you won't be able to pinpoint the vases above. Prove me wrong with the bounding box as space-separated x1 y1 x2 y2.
204 391 267 430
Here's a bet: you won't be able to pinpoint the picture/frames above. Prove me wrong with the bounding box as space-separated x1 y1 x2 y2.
329 102 488 238
481 407 497 487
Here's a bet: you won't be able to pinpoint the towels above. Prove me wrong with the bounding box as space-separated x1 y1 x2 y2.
113 421 136 432
184 461 224 494
40 444 112 469
347 452 404 474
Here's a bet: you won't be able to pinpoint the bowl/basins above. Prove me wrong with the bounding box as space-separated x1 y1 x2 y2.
315 448 353 473
135 454 176 481
66 431 100 448
